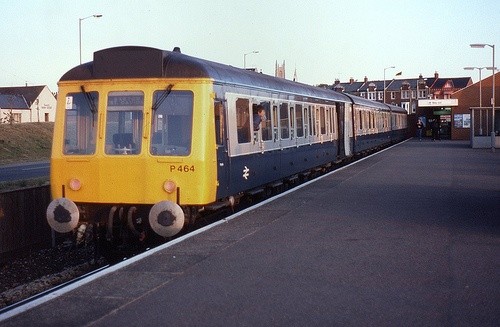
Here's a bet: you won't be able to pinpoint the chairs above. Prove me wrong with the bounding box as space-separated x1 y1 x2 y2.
113 133 134 147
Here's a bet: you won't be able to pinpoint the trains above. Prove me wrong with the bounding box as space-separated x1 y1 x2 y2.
46 45 410 240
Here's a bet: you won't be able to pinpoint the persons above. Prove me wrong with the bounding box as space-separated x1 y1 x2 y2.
431 119 440 142
416 119 424 142
239 105 268 142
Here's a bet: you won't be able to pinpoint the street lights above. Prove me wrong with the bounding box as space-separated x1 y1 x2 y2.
243 51 260 70
78 14 103 66
383 66 396 104
465 66 497 134
469 43 497 153
